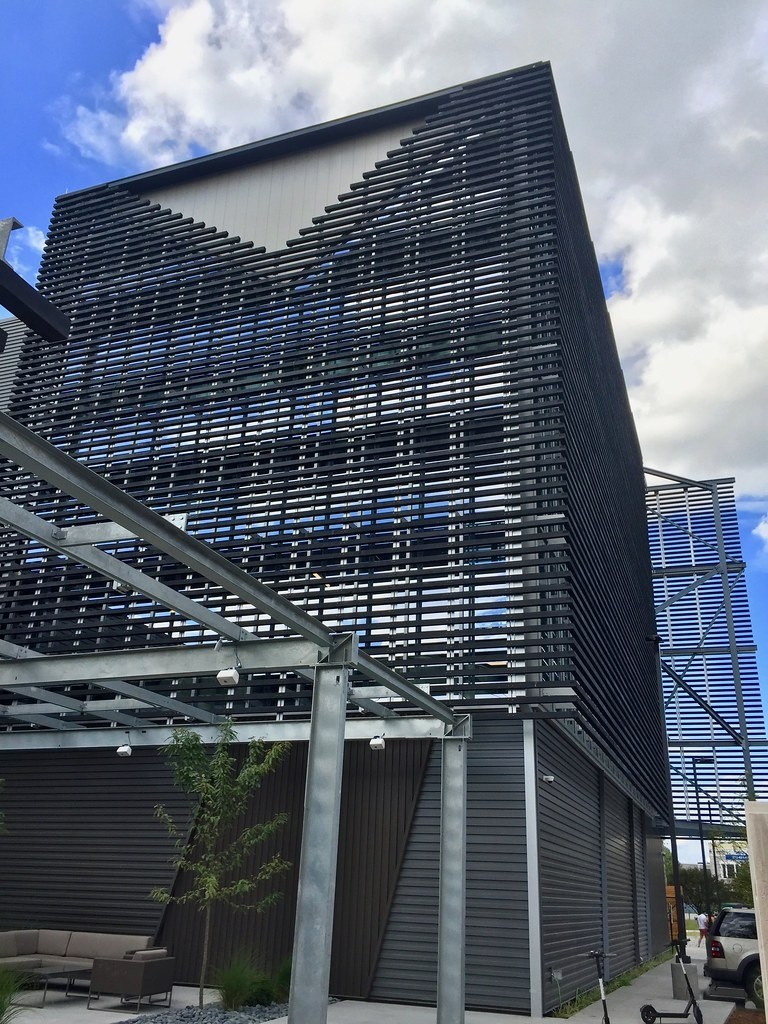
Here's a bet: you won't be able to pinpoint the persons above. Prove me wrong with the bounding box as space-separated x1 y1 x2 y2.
698 910 716 947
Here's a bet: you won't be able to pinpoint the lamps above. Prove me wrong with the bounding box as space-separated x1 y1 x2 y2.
113 579 133 595
217 668 240 685
116 745 132 756
369 734 385 750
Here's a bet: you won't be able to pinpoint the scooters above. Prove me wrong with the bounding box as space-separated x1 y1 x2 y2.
584 949 616 1024
639 937 705 1024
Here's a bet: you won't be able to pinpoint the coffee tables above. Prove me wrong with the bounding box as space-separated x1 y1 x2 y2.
8 966 100 1009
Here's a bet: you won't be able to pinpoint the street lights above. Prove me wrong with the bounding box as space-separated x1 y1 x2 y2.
690 756 724 927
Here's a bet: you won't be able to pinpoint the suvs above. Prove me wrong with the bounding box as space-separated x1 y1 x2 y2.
705 905 764 1008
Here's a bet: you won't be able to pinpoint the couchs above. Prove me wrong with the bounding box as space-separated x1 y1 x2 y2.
87 949 177 1014
0 929 168 984
